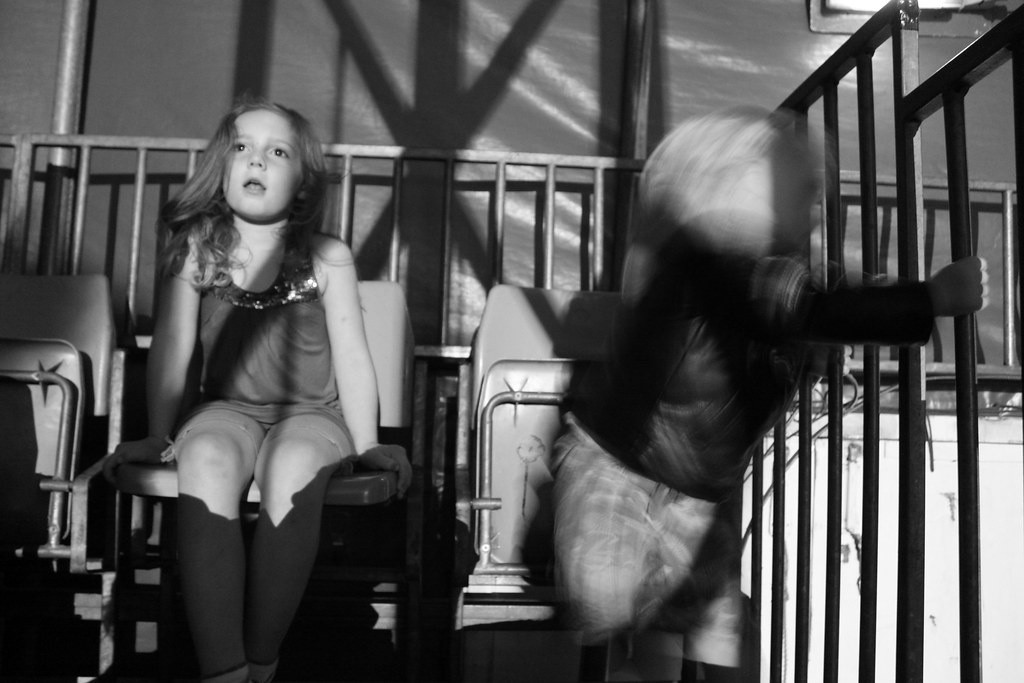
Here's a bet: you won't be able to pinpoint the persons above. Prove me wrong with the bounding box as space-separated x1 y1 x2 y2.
553 108 990 683
101 104 412 683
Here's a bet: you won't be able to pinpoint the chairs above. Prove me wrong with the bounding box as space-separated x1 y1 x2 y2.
462 284 713 681
112 277 431 683
0 273 125 682
